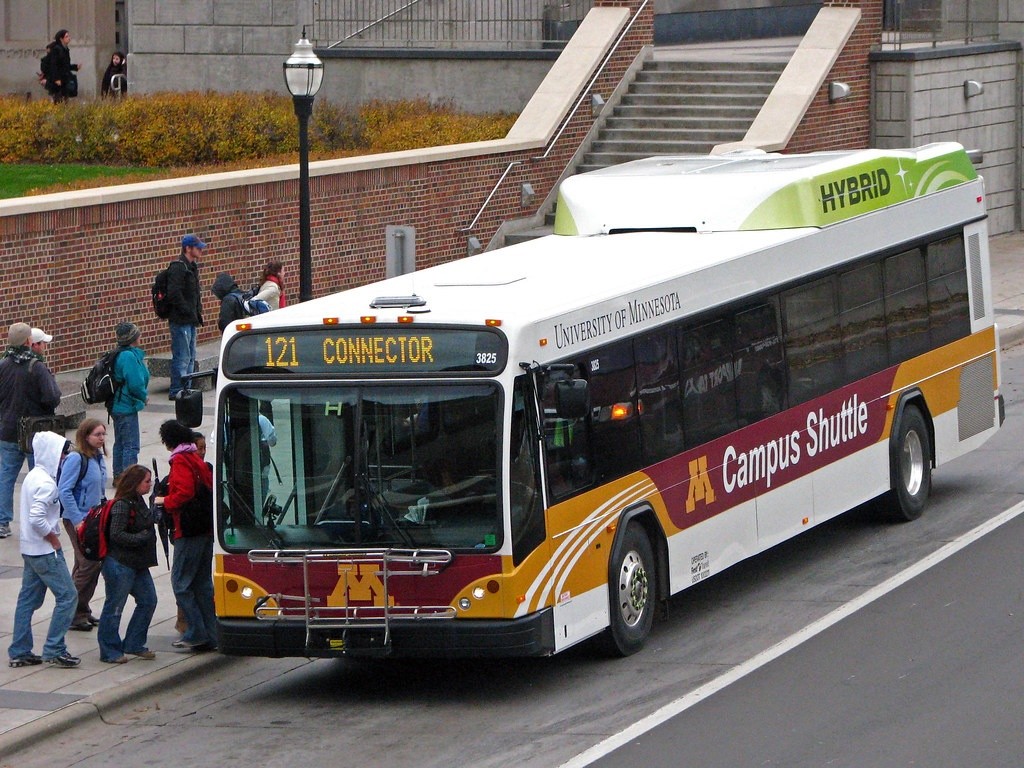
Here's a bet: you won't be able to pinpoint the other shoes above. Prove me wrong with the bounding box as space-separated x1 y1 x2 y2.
171 639 218 651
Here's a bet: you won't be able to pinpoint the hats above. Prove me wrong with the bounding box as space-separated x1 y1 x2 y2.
181 234 208 249
116 322 141 346
31 328 53 343
8 322 31 346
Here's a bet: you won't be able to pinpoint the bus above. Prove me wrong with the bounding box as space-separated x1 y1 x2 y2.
212 142 1005 659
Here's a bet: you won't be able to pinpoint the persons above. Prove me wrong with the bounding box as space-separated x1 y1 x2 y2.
99 464 157 664
222 402 277 528
7 431 82 669
212 273 258 368
155 419 219 652
57 420 106 632
0 323 62 541
330 489 382 544
107 322 150 488
167 235 208 400
40 29 82 105
251 261 285 310
535 334 690 496
101 52 127 101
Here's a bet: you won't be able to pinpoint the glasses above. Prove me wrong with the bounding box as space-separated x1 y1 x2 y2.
90 433 108 437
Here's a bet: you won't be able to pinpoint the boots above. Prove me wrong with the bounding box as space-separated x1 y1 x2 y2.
174 601 190 635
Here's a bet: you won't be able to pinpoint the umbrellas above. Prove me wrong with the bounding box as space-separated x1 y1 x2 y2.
151 459 170 571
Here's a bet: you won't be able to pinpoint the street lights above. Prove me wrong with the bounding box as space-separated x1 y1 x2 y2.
282 24 326 303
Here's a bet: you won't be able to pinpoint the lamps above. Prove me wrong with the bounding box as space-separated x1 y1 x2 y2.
592 92 605 118
467 237 482 257
965 78 984 101
520 182 535 208
829 80 852 103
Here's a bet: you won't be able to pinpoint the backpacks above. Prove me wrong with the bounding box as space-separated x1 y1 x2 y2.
226 290 273 319
152 269 168 319
80 347 132 405
252 285 261 297
76 497 119 561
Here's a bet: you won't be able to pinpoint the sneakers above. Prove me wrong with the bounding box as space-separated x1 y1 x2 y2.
68 618 100 632
43 651 81 668
113 650 155 663
8 651 43 668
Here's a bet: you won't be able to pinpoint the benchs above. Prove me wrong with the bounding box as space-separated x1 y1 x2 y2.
55 378 88 431
147 340 220 390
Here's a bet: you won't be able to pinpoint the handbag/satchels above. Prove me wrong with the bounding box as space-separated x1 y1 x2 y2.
179 480 229 539
18 383 54 415
150 476 177 530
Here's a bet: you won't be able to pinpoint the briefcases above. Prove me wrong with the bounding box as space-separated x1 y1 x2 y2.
18 414 66 454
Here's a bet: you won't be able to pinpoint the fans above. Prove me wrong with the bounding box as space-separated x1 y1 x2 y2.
262 495 283 528
